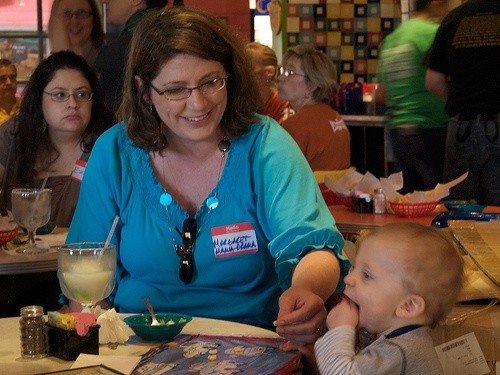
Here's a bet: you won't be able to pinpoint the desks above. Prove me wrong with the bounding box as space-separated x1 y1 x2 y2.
0 227 69 275
0 313 303 375
327 203 500 242
342 114 389 178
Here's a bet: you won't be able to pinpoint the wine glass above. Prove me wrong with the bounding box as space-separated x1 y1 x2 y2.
12 188 53 254
56 241 117 312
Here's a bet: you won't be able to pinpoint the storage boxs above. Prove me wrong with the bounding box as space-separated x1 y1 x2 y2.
45 321 101 361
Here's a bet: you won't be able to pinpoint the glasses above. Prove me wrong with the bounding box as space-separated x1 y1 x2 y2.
64 10 95 19
148 75 228 101
175 213 196 284
43 89 92 102
280 67 306 77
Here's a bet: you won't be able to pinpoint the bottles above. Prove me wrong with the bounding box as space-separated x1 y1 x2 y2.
19 306 49 360
373 189 387 215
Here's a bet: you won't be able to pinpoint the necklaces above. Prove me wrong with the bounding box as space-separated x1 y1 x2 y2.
85 46 93 61
156 126 231 257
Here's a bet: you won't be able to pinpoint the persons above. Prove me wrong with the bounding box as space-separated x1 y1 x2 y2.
0 57 22 128
374 0 463 197
244 42 296 126
0 49 116 229
277 222 468 375
421 0 500 207
58 6 353 347
48 0 184 113
279 45 352 171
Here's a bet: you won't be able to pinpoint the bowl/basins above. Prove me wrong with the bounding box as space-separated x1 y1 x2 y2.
444 200 485 214
21 222 55 235
123 314 192 341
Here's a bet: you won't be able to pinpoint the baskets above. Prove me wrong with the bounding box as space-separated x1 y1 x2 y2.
337 196 352 208
0 229 18 244
389 201 440 217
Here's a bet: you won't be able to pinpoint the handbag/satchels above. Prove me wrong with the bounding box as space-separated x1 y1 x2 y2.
447 219 500 303
